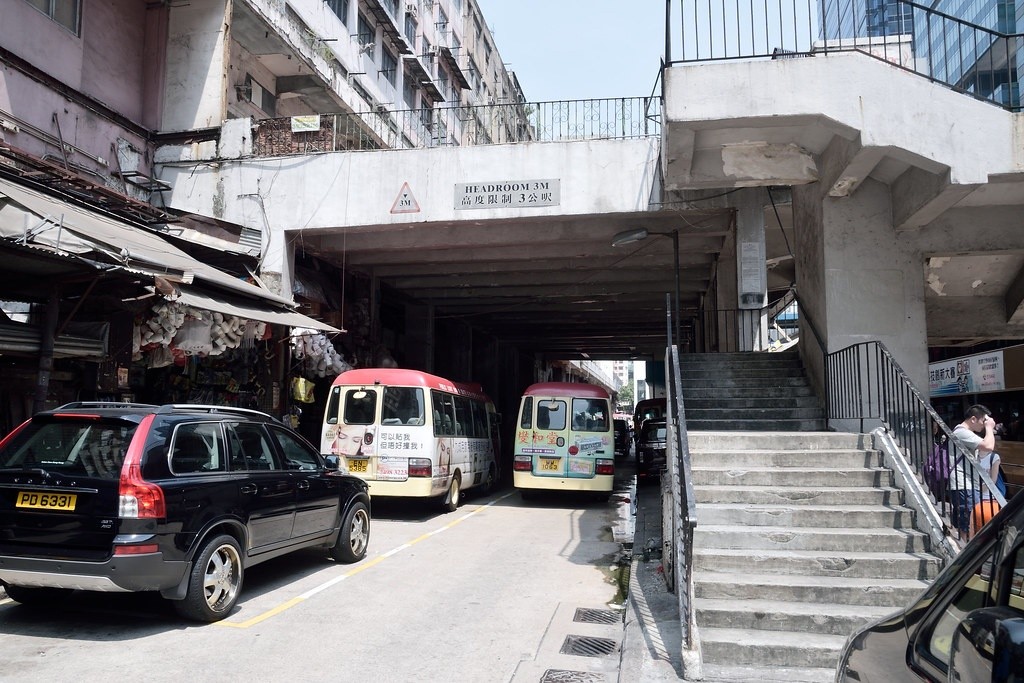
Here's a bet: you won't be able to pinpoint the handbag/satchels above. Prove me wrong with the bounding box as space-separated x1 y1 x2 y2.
293 375 315 403
990 454 1008 497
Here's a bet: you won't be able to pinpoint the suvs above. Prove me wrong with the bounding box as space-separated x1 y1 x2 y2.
0 401 372 626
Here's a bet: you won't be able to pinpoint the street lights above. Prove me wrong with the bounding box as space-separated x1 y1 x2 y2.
611 228 680 366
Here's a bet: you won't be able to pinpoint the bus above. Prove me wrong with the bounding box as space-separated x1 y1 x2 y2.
631 398 665 445
319 368 502 512
512 382 615 505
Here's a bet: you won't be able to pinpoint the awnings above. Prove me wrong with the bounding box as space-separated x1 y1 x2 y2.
118 268 348 376
0 174 300 337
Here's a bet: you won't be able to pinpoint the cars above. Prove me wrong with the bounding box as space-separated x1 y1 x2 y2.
612 419 633 455
832 480 1024 683
634 417 667 482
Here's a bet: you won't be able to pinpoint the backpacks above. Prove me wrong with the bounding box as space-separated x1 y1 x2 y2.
924 426 967 498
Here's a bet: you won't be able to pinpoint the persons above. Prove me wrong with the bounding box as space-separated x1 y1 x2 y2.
931 406 1006 543
